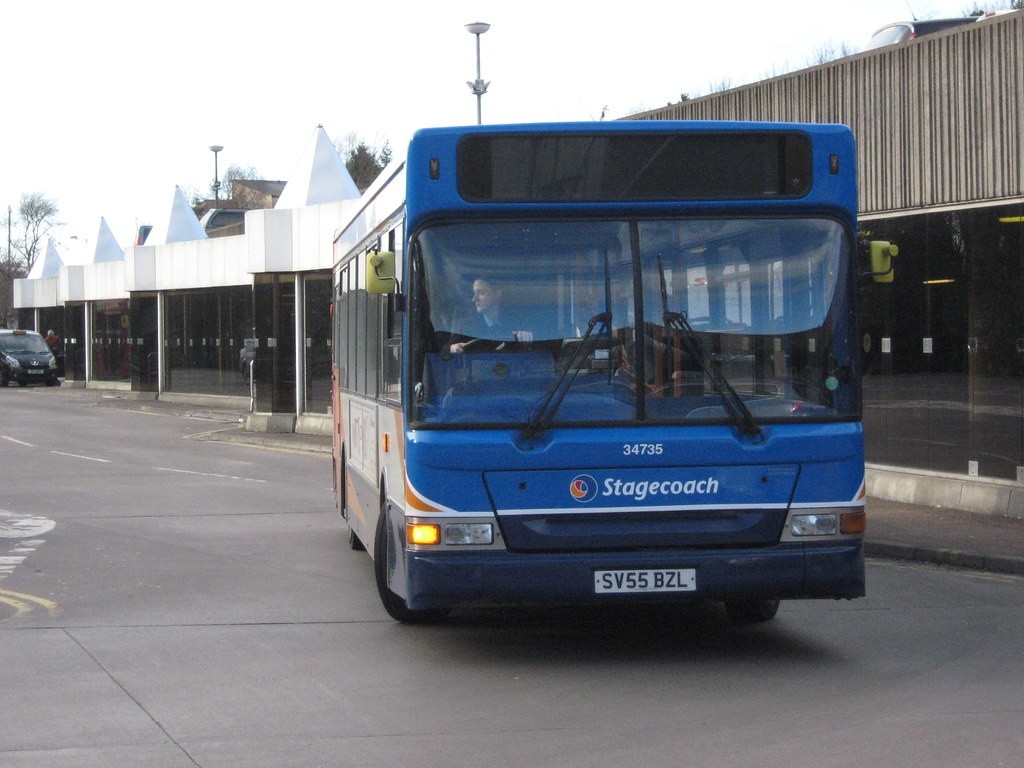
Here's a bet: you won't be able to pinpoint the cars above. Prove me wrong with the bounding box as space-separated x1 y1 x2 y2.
0 329 58 387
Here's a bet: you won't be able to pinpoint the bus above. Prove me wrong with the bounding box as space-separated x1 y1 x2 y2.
331 120 901 625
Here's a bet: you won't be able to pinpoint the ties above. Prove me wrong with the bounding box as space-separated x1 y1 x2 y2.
490 324 501 339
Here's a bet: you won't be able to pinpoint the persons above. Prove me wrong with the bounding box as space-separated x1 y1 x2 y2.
440 271 544 360
45 329 63 368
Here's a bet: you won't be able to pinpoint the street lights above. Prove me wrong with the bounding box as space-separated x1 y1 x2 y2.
464 22 490 125
210 145 224 208
